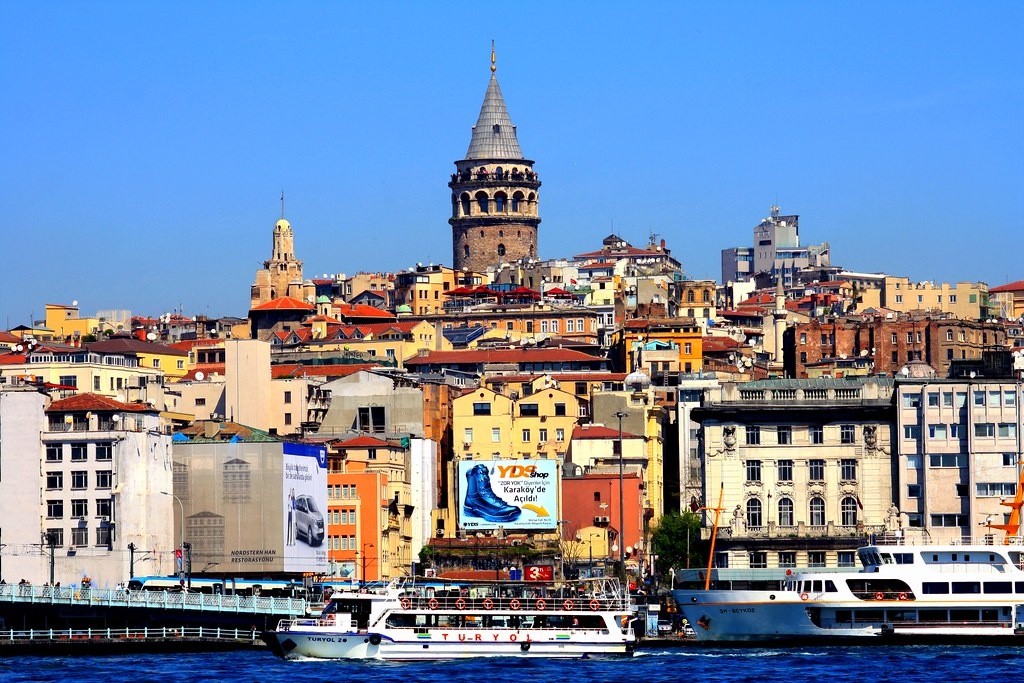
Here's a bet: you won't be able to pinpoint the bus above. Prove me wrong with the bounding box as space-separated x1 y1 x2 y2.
127 576 472 602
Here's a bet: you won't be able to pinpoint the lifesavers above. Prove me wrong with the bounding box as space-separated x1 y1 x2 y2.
591 599 599 610
429 598 439 609
899 593 908 600
456 599 466 609
401 598 411 609
564 600 573 610
536 599 546 610
327 614 335 626
801 593 809 600
520 641 532 651
369 633 382 645
509 599 521 610
875 592 885 600
484 599 493 610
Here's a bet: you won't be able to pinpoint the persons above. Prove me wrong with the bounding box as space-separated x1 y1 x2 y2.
632 579 648 605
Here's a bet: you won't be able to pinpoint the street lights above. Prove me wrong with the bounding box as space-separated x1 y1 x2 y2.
611 412 630 581
581 533 600 577
160 491 184 577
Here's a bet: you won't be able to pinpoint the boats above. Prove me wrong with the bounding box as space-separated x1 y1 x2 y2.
669 483 1023 649
259 575 636 662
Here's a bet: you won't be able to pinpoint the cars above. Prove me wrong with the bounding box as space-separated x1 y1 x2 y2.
294 494 326 547
